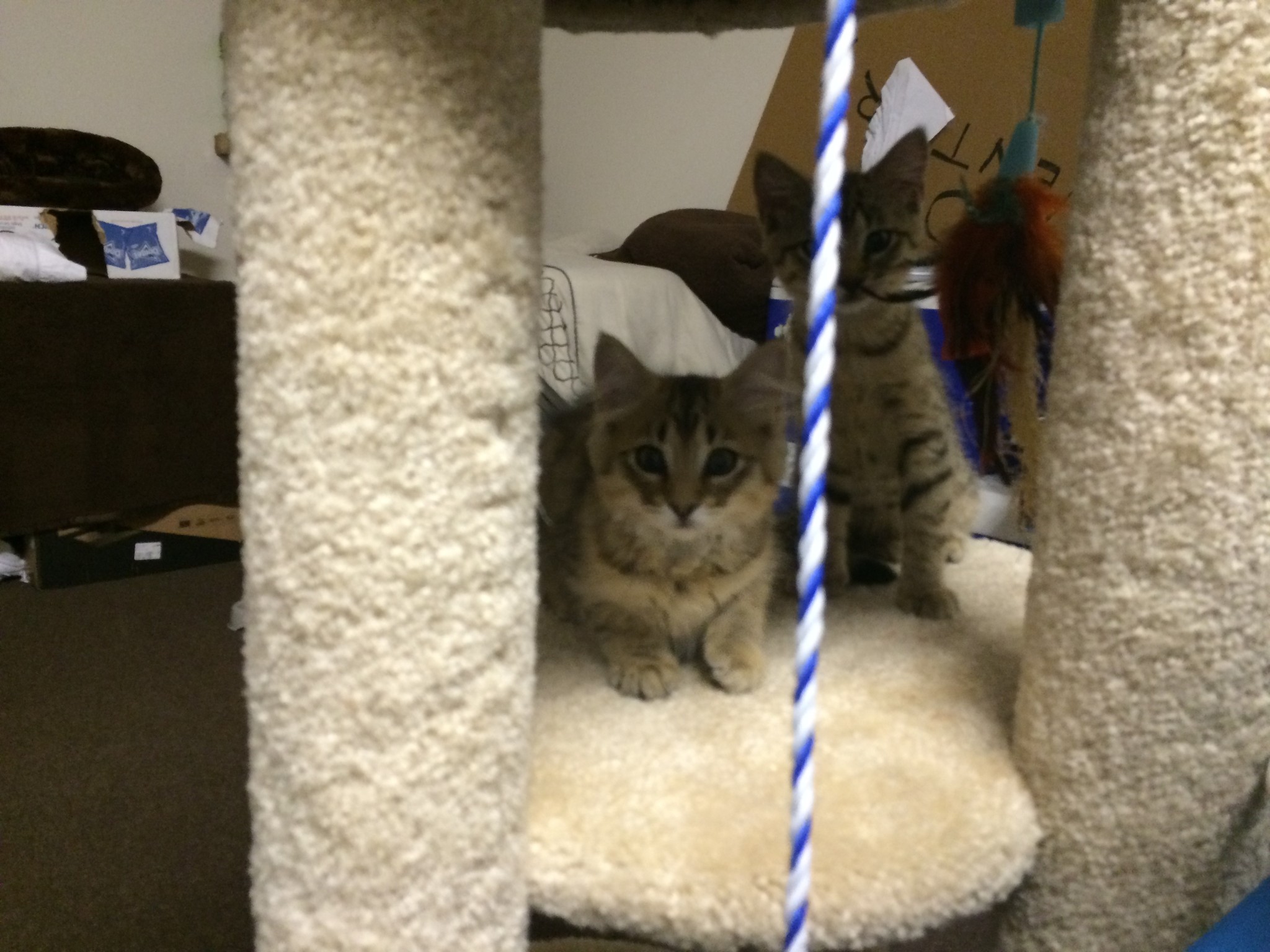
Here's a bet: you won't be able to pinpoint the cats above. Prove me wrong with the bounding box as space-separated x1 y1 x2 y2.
536 325 798 707
745 120 978 624
